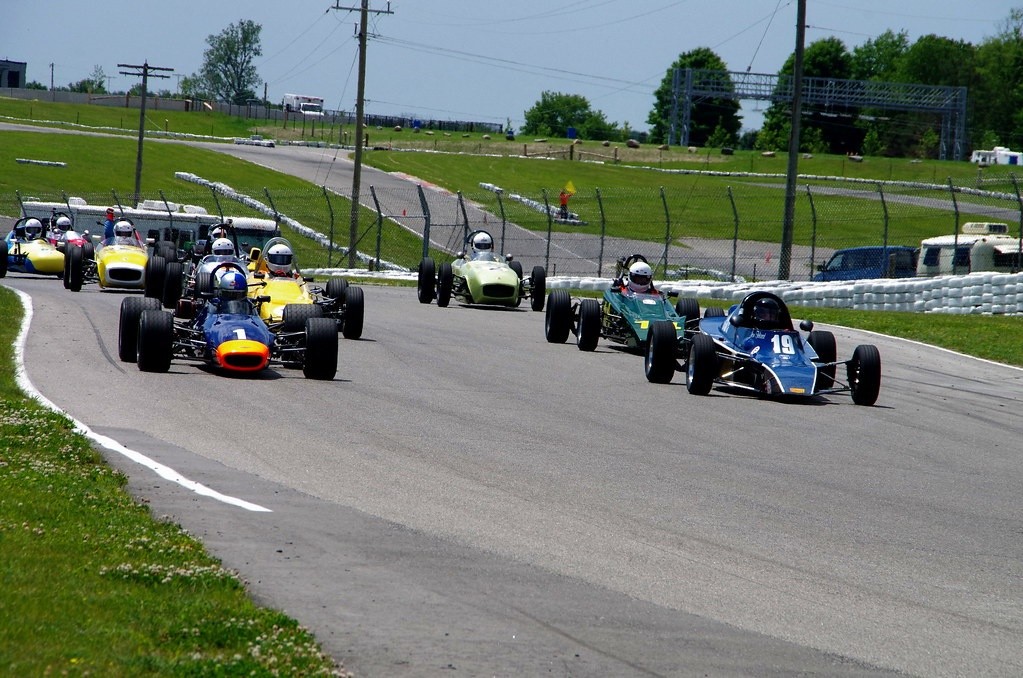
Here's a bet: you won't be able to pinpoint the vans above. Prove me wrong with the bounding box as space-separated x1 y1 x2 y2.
813 246 916 282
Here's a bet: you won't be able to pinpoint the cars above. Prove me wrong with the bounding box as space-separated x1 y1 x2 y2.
418 230 545 312
545 258 726 356
145 224 364 334
0 212 94 277
64 236 176 293
118 261 338 381
647 291 882 405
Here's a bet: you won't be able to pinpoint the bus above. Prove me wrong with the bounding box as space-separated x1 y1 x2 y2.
19 195 281 257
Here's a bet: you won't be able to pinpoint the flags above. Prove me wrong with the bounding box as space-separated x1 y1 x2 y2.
563 179 577 196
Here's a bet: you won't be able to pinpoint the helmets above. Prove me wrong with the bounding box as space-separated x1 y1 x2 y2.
113 220 133 244
56 216 71 233
25 218 43 239
211 227 227 242
212 237 235 262
472 232 492 253
750 297 782 328
628 261 652 292
220 271 247 301
267 243 293 273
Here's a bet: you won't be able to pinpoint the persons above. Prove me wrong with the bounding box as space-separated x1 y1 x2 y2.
55 216 74 235
22 218 47 244
204 222 227 255
749 296 784 329
206 238 239 262
111 220 137 246
265 243 298 281
469 231 496 261
559 187 574 220
103 207 117 245
617 261 660 299
212 269 251 316
